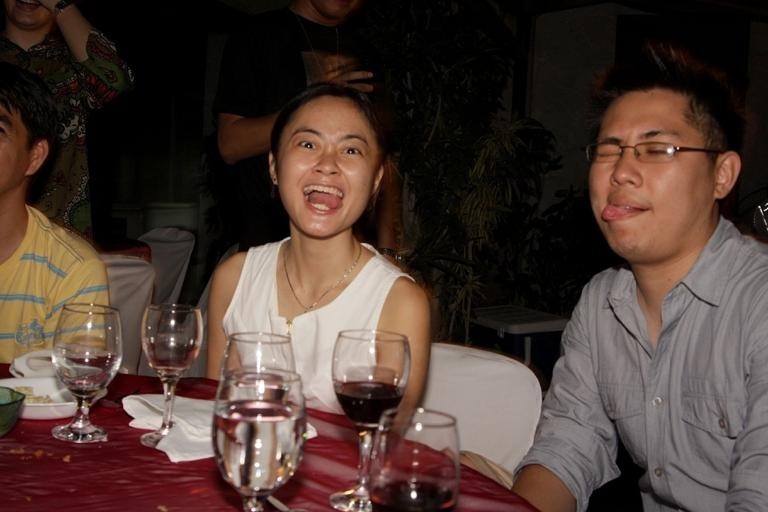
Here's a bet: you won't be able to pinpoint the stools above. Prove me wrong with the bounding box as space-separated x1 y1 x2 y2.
468 303 570 365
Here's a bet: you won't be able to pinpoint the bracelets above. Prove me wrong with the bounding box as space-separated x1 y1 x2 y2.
378 247 397 258
54 1 73 15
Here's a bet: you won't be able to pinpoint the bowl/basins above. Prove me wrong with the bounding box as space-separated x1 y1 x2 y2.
0 388 24 435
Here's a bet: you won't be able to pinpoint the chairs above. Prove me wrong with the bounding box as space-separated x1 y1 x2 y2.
138 228 196 306
418 343 543 470
97 254 156 374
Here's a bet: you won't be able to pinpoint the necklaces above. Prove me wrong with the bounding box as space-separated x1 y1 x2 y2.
281 234 362 334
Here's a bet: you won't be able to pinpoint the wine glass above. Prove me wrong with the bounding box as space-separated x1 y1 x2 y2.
50 297 124 445
327 327 410 512
138 301 203 451
209 367 308 512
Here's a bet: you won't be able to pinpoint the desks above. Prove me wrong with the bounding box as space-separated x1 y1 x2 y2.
86 236 153 262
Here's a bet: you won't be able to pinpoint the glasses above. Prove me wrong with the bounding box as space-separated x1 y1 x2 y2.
585 139 729 167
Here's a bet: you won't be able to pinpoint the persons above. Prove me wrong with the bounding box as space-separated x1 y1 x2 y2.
509 56 768 511
214 0 399 262
0 60 111 364
205 82 433 437
1 0 135 246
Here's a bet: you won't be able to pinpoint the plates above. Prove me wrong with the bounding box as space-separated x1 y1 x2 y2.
1 375 110 422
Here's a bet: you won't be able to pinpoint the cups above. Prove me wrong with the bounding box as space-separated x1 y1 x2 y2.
362 405 460 512
217 330 299 402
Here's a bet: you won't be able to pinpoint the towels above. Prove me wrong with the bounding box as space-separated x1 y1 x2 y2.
121 394 318 465
